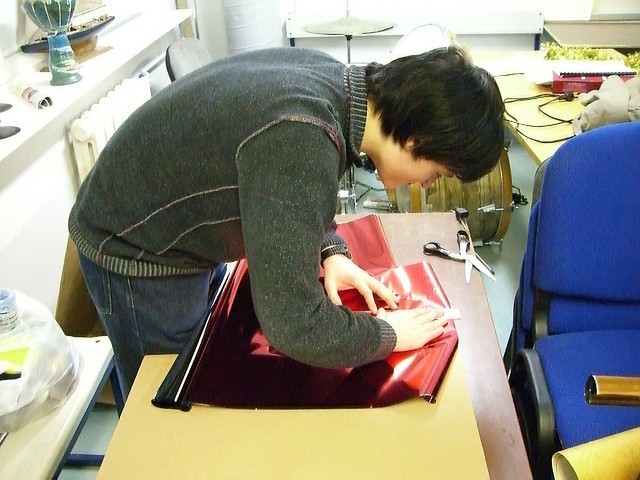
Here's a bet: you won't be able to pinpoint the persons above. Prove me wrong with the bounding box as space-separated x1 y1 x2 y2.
65 44 502 393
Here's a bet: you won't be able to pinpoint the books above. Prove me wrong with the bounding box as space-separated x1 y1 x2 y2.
544 60 638 78
552 72 637 93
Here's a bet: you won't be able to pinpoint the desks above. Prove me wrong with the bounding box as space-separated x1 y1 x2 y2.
0 335 126 480
470 50 639 167
225 213 534 480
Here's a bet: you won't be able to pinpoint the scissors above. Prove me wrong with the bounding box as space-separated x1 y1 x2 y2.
423 230 496 284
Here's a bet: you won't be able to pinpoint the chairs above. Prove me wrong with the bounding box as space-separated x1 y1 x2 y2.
164 36 213 82
501 122 640 480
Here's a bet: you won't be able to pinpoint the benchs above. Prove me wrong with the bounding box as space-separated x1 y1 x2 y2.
285 11 544 50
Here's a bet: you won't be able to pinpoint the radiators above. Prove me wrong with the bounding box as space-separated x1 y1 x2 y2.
69 76 151 185
